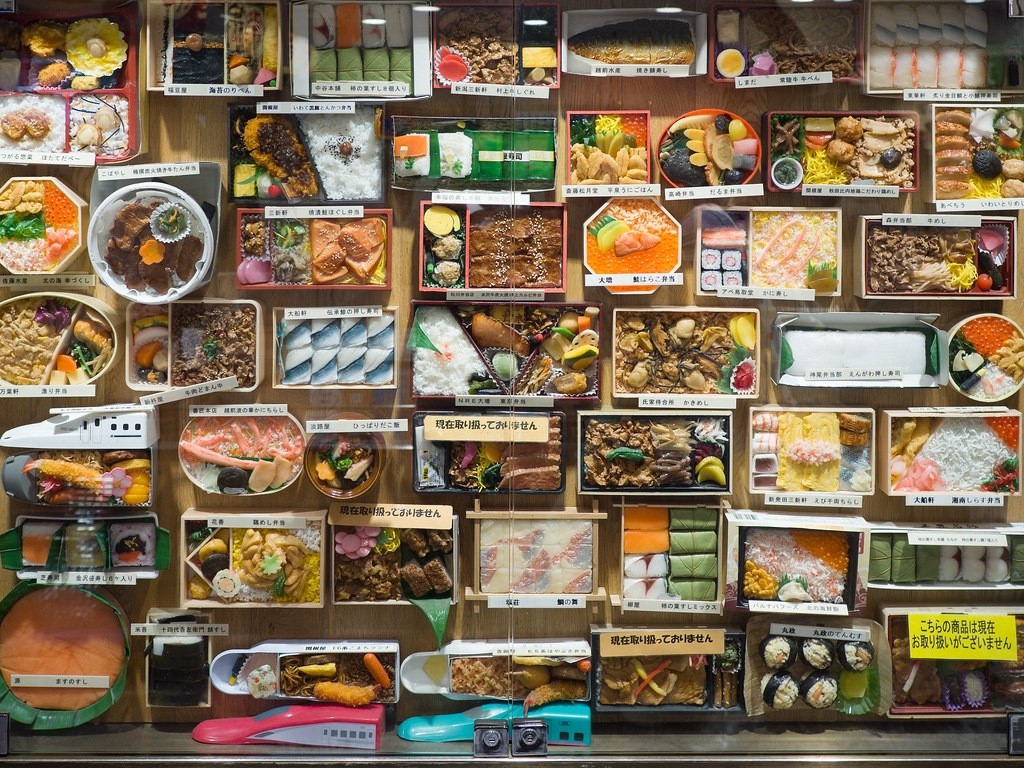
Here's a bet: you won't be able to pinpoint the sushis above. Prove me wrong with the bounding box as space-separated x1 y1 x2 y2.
0 1 1024 767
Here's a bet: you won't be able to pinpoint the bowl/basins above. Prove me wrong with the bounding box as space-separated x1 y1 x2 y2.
0 0 1024 730
